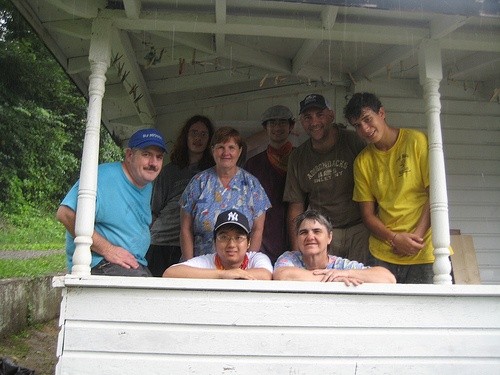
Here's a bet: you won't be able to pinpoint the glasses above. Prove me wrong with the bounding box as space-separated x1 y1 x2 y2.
216 234 246 243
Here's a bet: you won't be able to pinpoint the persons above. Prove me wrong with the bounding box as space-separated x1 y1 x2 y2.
283 94 379 269
273 211 398 286
343 92 455 284
180 127 272 263
56 130 169 277
145 114 216 278
244 105 309 267
162 209 272 280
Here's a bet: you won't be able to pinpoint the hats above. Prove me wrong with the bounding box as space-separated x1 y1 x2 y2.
260 106 295 124
299 94 327 115
128 129 168 153
214 209 250 235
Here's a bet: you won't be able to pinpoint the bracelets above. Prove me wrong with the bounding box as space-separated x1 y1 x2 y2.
393 232 397 241
